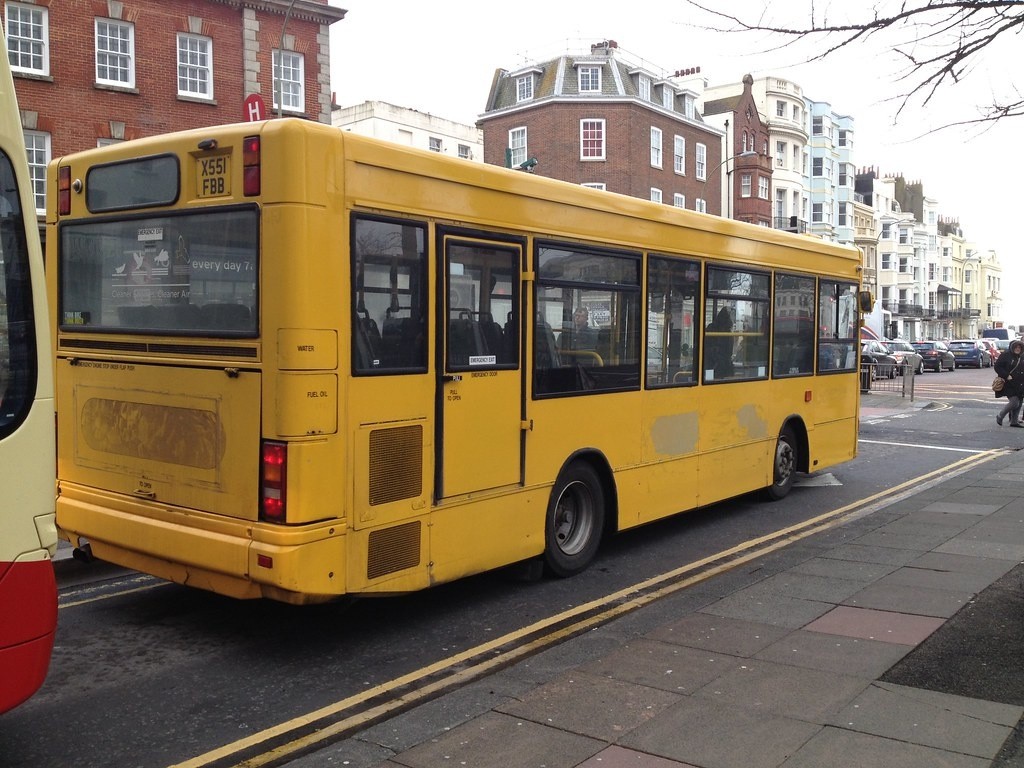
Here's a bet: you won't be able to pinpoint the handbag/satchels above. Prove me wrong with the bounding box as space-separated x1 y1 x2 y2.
991 377 1005 391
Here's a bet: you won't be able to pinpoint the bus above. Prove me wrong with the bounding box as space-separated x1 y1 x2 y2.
44 116 863 612
1 19 61 717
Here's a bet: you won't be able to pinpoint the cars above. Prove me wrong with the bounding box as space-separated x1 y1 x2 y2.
860 339 897 381
982 340 1002 366
995 340 1011 354
880 340 925 374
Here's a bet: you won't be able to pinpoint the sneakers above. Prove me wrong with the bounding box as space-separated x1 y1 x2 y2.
996 415 1003 425
1010 423 1023 428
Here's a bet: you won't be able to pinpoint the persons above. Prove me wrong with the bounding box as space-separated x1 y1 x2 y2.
993 336 1024 428
554 306 598 365
697 305 736 378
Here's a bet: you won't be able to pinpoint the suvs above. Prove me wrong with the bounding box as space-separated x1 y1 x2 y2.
947 340 991 368
909 340 956 372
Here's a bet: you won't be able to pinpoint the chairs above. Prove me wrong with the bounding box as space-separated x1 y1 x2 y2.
116 301 561 372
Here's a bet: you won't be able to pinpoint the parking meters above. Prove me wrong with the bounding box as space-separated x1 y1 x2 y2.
875 218 918 300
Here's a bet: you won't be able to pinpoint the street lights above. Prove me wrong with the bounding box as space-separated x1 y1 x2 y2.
699 151 755 213
959 249 982 339
